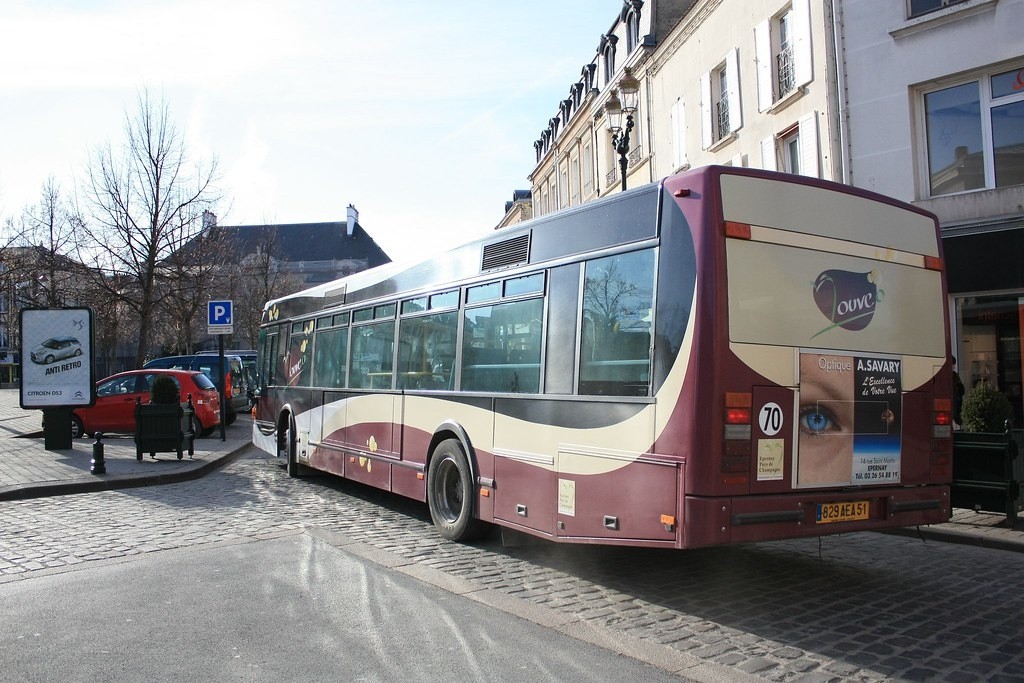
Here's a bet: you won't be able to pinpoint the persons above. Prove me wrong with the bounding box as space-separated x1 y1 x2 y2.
949 354 967 432
799 354 854 485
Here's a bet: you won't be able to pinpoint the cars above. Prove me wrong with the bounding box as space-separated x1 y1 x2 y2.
71 369 220 439
31 336 83 364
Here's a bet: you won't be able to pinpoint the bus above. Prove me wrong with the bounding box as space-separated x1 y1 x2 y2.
252 165 956 551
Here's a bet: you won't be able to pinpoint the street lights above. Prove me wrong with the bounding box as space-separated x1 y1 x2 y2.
603 65 641 191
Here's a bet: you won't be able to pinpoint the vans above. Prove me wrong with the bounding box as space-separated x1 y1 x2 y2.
142 354 249 426
